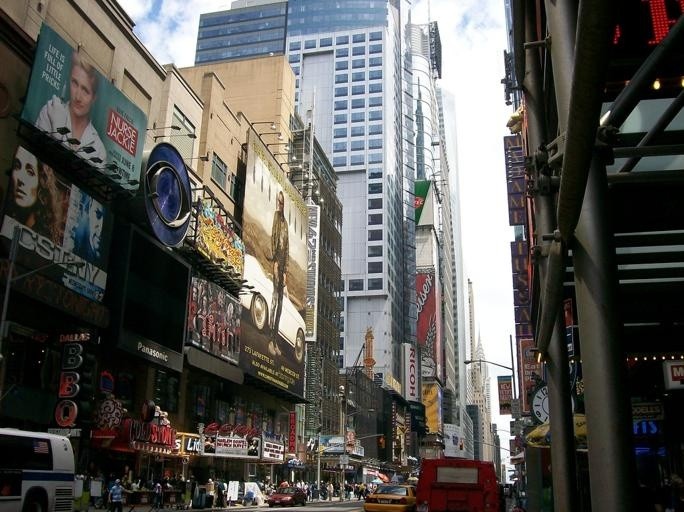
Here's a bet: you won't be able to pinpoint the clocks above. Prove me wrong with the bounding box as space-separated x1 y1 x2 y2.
528 384 578 426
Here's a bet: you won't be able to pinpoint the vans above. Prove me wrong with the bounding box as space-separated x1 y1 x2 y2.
414 456 499 512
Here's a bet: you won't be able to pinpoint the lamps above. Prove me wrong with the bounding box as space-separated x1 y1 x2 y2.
43 126 159 204
147 125 208 164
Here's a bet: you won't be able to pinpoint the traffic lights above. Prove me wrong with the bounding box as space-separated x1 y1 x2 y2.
378 435 386 449
459 441 463 451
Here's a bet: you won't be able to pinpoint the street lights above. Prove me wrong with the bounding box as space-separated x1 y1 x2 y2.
464 358 525 493
340 408 375 502
0 260 86 348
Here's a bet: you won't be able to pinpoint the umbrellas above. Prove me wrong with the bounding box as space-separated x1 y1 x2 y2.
372 478 384 484
407 476 418 481
378 472 389 483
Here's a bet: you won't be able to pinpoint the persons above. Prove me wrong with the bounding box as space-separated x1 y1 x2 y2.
208 478 228 508
105 475 185 512
186 277 240 360
71 189 108 272
248 438 258 456
344 482 373 501
32 52 106 172
205 436 214 452
279 479 341 502
266 190 289 356
4 147 61 247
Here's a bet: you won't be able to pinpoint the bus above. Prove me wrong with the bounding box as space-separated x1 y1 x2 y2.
0 425 75 512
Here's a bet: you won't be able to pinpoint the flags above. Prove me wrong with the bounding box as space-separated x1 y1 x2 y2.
33 441 48 453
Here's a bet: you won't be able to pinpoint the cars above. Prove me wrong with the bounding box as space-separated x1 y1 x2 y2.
266 486 308 507
363 483 418 512
